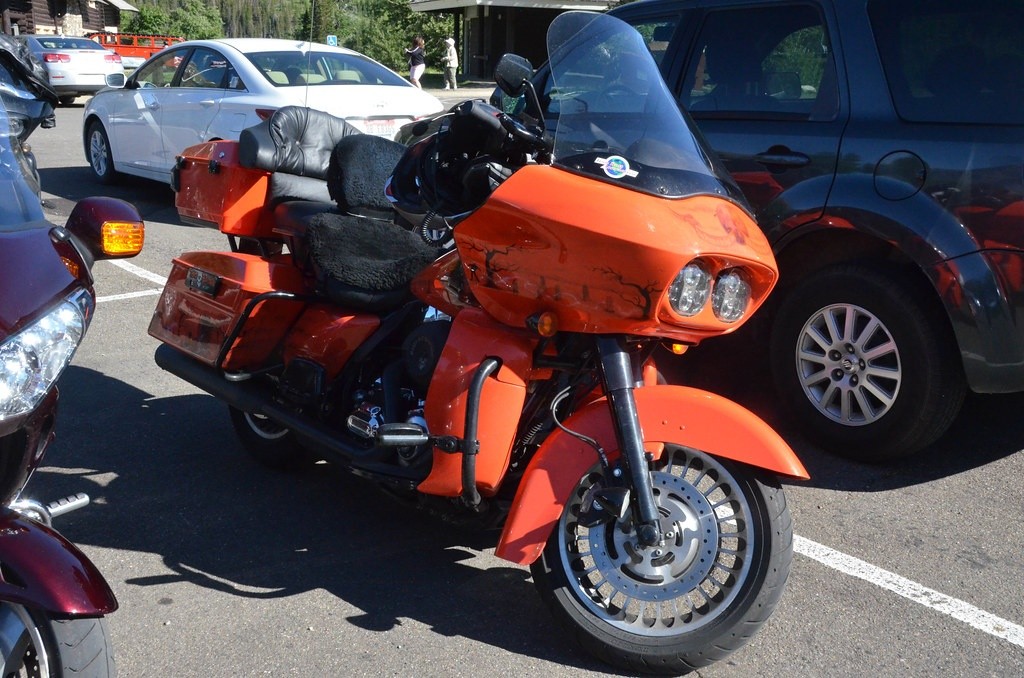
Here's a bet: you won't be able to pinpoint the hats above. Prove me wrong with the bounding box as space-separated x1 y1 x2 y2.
445 38 456 46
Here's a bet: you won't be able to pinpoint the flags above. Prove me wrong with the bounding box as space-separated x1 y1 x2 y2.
209 60 227 69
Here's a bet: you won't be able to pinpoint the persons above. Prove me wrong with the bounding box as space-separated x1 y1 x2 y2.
441 37 459 91
404 37 426 89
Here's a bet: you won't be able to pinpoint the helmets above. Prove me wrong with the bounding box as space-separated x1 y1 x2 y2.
384 99 530 230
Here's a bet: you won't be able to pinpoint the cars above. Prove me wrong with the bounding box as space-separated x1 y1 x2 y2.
83 38 445 185
16 34 124 104
487 0 1024 462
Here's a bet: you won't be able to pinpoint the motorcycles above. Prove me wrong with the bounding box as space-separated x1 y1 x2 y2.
0 30 59 222
0 94 145 678
146 11 810 675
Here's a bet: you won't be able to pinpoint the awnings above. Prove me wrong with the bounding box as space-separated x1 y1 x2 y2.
102 0 139 13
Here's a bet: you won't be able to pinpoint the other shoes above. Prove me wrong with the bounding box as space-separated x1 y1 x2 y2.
443 85 458 91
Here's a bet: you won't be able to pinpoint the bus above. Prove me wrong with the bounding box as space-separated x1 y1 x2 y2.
84 31 189 69
